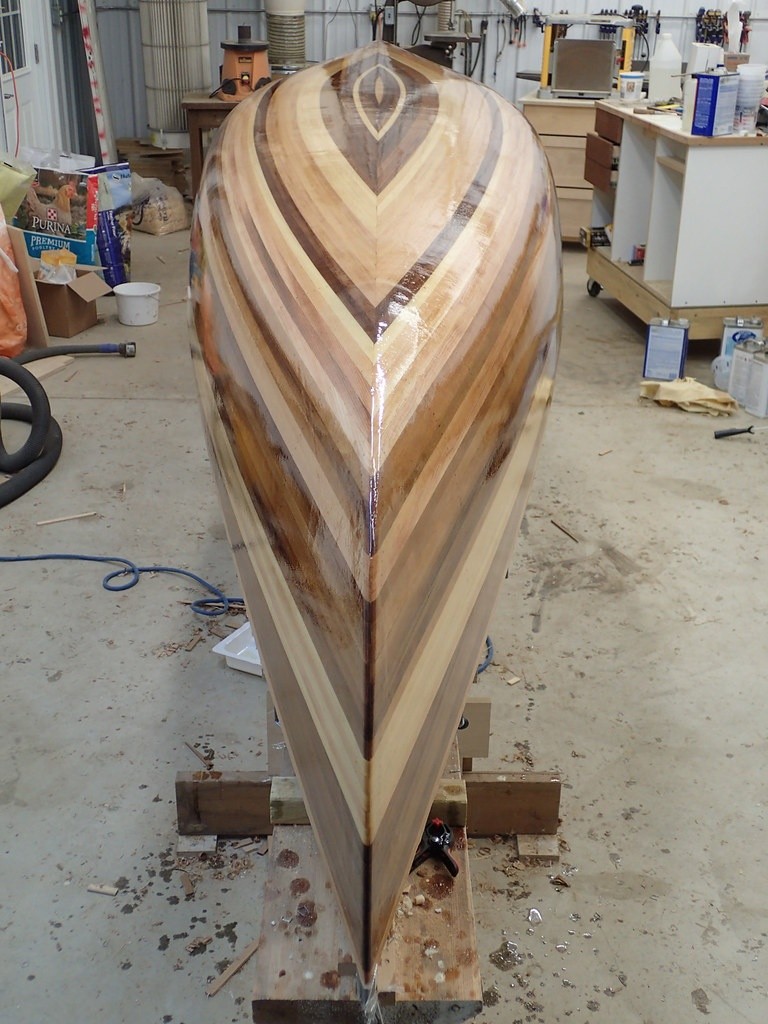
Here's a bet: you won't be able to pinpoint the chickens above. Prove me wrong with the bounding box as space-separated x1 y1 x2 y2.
26 181 79 236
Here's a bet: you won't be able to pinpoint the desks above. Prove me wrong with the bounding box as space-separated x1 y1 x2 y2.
183 90 252 202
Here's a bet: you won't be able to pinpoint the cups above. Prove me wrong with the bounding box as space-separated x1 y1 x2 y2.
734 63 766 135
619 73 644 101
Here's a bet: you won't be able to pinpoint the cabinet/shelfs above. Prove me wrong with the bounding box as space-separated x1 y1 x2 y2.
577 95 768 343
517 85 647 244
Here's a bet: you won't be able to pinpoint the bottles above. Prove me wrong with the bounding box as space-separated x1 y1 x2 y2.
649 33 682 105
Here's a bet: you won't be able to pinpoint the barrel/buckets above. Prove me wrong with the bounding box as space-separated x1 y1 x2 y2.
113 282 163 326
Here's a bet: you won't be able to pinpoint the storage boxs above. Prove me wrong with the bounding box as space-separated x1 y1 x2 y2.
28 255 114 339
579 227 612 248
689 71 740 136
